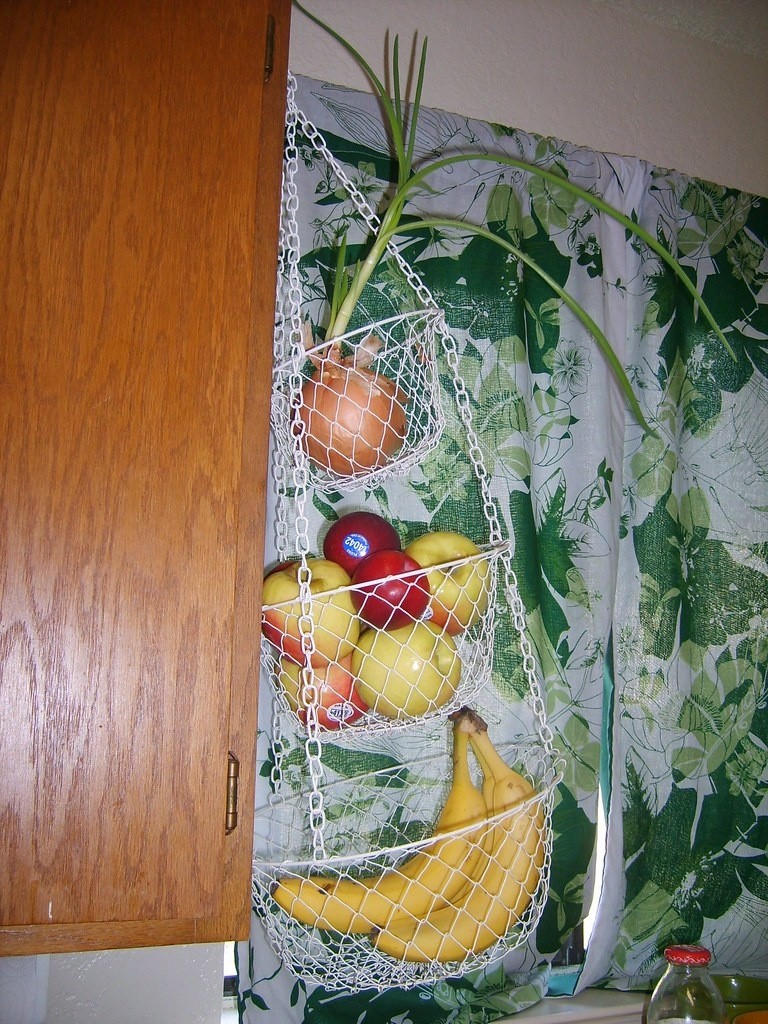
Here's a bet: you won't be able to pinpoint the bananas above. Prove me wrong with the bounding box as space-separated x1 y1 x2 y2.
271 712 542 961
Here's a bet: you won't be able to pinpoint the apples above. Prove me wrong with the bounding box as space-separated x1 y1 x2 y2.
262 512 490 727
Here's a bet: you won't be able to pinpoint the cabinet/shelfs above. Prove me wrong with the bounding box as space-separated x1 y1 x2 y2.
0 0 293 963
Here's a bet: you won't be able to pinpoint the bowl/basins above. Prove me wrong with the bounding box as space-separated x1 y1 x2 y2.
653 975 768 1024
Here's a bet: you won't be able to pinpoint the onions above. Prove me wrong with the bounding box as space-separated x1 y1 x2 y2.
291 367 403 473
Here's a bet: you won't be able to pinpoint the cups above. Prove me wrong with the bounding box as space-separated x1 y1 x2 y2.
732 1010 768 1024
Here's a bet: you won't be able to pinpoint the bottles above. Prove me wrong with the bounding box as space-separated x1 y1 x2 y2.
646 946 726 1024
